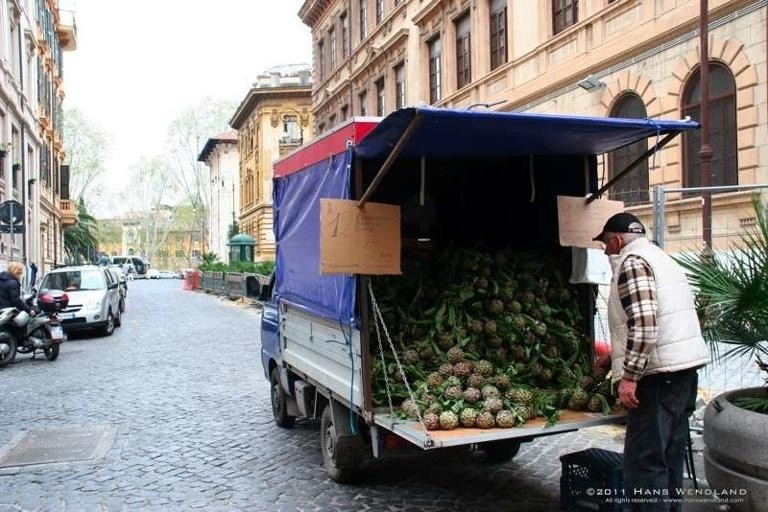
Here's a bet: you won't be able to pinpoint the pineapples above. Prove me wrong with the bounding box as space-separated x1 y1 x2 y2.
368 247 611 428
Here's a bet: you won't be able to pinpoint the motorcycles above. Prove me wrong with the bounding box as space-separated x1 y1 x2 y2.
0 288 69 368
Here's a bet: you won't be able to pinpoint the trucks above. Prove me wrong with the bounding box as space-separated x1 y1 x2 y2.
262 107 700 484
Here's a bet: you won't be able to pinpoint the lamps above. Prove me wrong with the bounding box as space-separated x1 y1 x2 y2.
578 76 606 93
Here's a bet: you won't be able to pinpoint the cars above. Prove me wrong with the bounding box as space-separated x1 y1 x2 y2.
38 265 125 335
156 271 178 279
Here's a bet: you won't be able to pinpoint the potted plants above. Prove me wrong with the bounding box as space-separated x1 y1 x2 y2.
672 191 768 512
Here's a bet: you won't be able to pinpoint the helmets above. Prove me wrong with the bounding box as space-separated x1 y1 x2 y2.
11 310 30 327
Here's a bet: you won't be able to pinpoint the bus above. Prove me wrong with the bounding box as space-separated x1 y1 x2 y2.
109 256 147 278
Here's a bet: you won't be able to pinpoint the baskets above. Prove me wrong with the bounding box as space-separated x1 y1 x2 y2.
560 448 626 511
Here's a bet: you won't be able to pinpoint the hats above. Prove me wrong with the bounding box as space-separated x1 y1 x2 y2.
592 213 645 241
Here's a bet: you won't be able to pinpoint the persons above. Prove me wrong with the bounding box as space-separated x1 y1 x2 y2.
0 262 35 317
127 266 134 280
592 211 710 512
